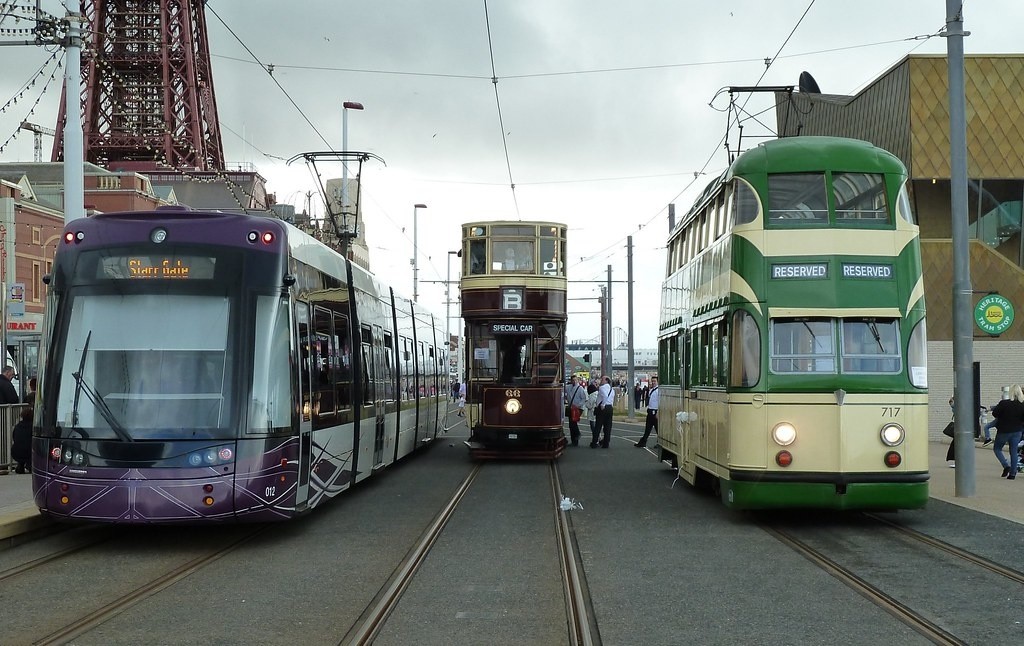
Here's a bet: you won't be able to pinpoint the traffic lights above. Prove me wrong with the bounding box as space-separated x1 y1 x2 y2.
582 354 590 363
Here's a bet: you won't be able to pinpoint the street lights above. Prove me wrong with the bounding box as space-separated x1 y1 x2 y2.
412 203 428 305
341 99 364 254
445 249 459 337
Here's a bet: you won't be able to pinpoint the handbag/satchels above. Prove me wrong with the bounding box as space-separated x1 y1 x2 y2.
593 407 598 416
571 405 580 421
565 406 570 417
943 421 954 437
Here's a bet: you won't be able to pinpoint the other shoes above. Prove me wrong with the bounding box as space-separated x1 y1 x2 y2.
15 466 25 474
634 444 646 447
983 438 992 447
1002 466 1010 477
1007 475 1015 479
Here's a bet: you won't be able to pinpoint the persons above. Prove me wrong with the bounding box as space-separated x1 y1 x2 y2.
0 367 37 475
634 383 648 410
585 385 604 446
949 397 955 468
982 384 1024 480
472 258 486 274
612 379 628 396
449 378 467 417
565 375 586 447
590 376 615 448
502 248 519 270
634 376 659 449
587 378 600 388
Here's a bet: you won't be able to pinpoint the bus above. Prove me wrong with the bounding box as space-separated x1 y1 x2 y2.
659 84 933 512
461 219 576 460
32 151 449 523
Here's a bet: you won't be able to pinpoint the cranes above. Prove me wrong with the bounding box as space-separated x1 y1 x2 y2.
20 120 56 162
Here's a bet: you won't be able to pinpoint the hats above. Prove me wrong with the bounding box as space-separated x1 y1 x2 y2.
21 409 33 417
